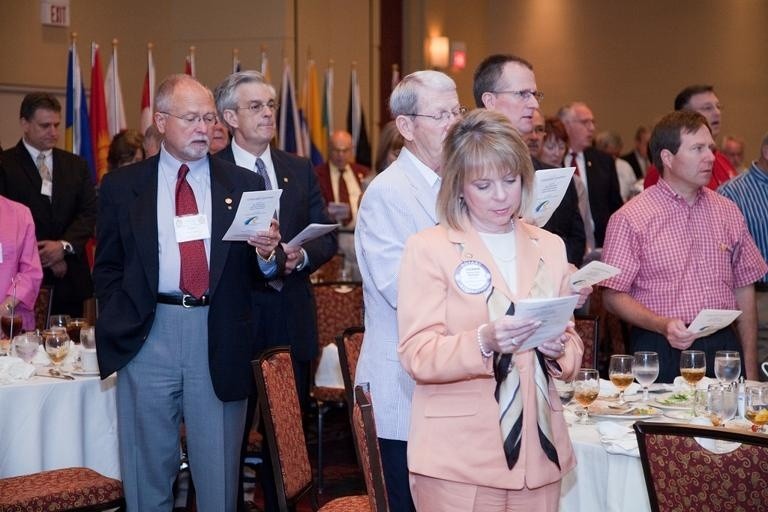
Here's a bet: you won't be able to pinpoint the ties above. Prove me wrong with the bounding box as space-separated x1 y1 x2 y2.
38 152 52 197
255 159 278 222
336 168 355 228
569 153 582 175
173 165 209 298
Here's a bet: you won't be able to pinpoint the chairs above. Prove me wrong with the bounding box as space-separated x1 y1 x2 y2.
83 298 99 326
0 467 124 512
33 287 53 331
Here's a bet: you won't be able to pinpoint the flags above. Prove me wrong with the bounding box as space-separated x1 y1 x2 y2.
67 40 269 273
276 55 371 163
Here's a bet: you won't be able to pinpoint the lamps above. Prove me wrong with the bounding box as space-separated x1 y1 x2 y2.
429 37 450 69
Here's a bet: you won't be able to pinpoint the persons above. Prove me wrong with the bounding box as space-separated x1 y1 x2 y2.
312 129 373 234
2 54 229 336
92 70 338 511
353 55 624 510
594 81 768 382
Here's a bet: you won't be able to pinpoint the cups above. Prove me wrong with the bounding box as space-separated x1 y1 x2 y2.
309 265 355 294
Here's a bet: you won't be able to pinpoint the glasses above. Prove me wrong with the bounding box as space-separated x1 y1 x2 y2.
230 102 276 113
403 107 464 124
495 88 545 104
163 112 220 127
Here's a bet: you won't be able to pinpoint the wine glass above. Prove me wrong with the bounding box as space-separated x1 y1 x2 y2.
0 310 100 380
553 349 768 434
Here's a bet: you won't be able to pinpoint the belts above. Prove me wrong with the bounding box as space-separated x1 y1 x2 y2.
157 292 211 308
